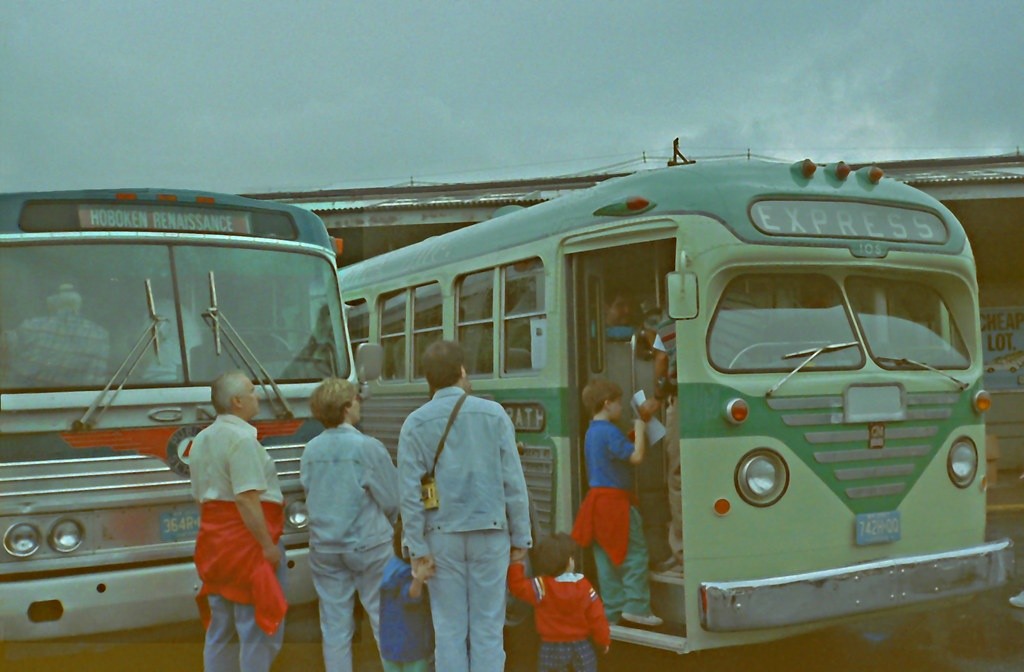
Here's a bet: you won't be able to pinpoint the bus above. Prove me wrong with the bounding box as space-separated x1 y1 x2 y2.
311 159 1014 654
0 189 384 642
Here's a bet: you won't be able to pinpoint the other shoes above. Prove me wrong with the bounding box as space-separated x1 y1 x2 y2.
651 557 683 577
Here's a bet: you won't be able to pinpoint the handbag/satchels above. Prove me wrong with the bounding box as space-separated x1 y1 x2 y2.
420 478 437 511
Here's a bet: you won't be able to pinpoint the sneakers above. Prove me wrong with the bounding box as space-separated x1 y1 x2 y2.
608 613 662 627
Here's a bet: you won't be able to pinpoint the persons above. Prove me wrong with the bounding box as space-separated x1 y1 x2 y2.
603 288 639 341
397 340 534 672
0 278 110 388
570 381 664 626
299 376 397 672
188 370 289 672
508 531 611 672
638 267 685 578
